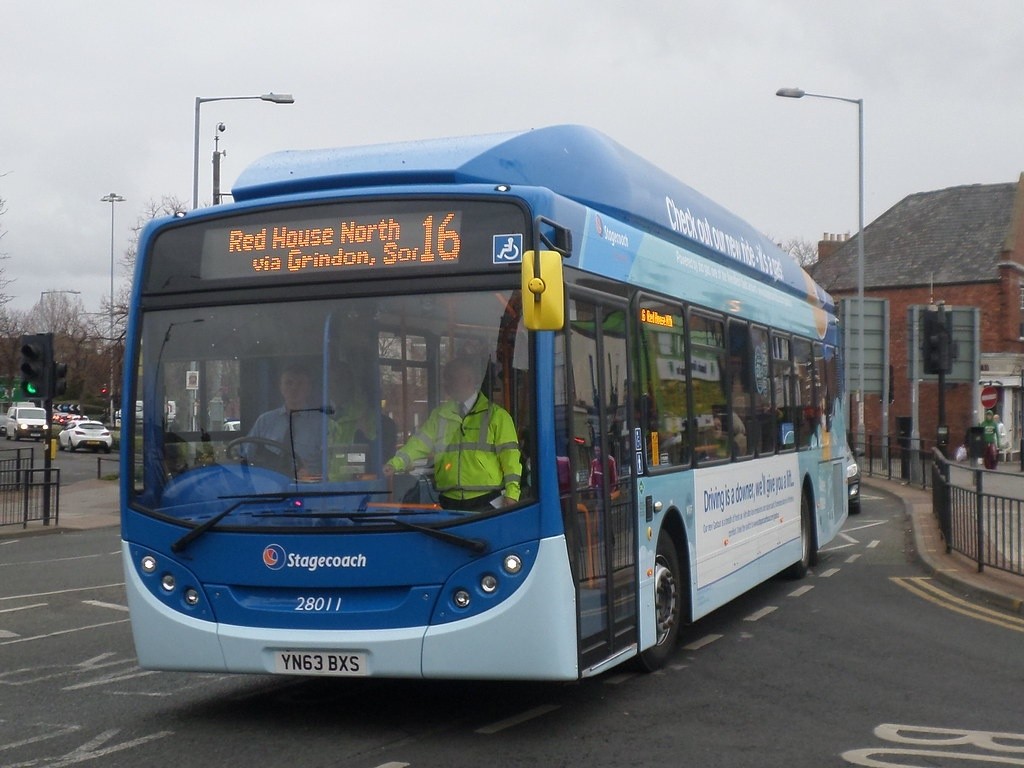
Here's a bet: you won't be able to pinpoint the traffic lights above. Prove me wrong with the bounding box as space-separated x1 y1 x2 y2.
19 332 49 401
929 330 950 370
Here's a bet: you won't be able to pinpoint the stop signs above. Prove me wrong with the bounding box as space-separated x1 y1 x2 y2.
981 386 998 409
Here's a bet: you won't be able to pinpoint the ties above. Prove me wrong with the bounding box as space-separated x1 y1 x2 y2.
459 402 465 418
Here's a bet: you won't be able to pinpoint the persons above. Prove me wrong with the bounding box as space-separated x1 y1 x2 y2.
696 414 729 462
330 366 397 475
557 445 618 549
980 410 1000 470
236 361 341 478
382 360 522 511
993 414 1006 464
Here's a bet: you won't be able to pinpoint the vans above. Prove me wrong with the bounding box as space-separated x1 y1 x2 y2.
6 406 47 442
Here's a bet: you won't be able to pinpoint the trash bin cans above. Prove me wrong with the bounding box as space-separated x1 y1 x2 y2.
966 426 985 465
896 416 912 480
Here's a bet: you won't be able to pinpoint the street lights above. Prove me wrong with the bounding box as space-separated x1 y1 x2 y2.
193 92 295 210
100 192 126 428
776 87 867 472
40 290 82 307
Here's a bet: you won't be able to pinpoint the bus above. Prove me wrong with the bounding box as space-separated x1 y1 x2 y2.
114 125 849 683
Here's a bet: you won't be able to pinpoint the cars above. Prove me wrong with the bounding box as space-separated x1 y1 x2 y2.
115 400 241 433
0 407 8 438
846 443 867 514
57 419 113 454
